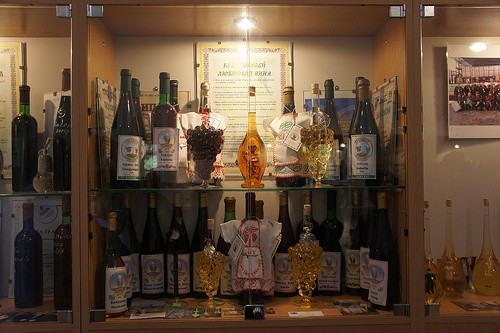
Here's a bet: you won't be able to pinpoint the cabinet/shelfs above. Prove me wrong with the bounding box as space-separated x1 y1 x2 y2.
0 0 500 333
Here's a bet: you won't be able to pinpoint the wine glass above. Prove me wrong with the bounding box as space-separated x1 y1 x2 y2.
287 247 322 306
187 129 224 188
193 250 225 308
299 126 334 188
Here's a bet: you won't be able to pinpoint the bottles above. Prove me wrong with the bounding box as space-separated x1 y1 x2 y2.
170 79 178 114
350 79 381 185
10 83 39 193
131 77 146 188
274 86 303 186
321 79 344 185
54 195 71 311
13 202 44 307
423 201 443 304
472 198 500 296
198 83 211 113
97 190 401 318
151 71 178 186
309 83 320 114
110 69 140 189
52 68 71 193
238 85 266 188
436 200 467 299
345 76 364 185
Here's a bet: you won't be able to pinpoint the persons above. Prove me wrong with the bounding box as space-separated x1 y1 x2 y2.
455 78 500 111
